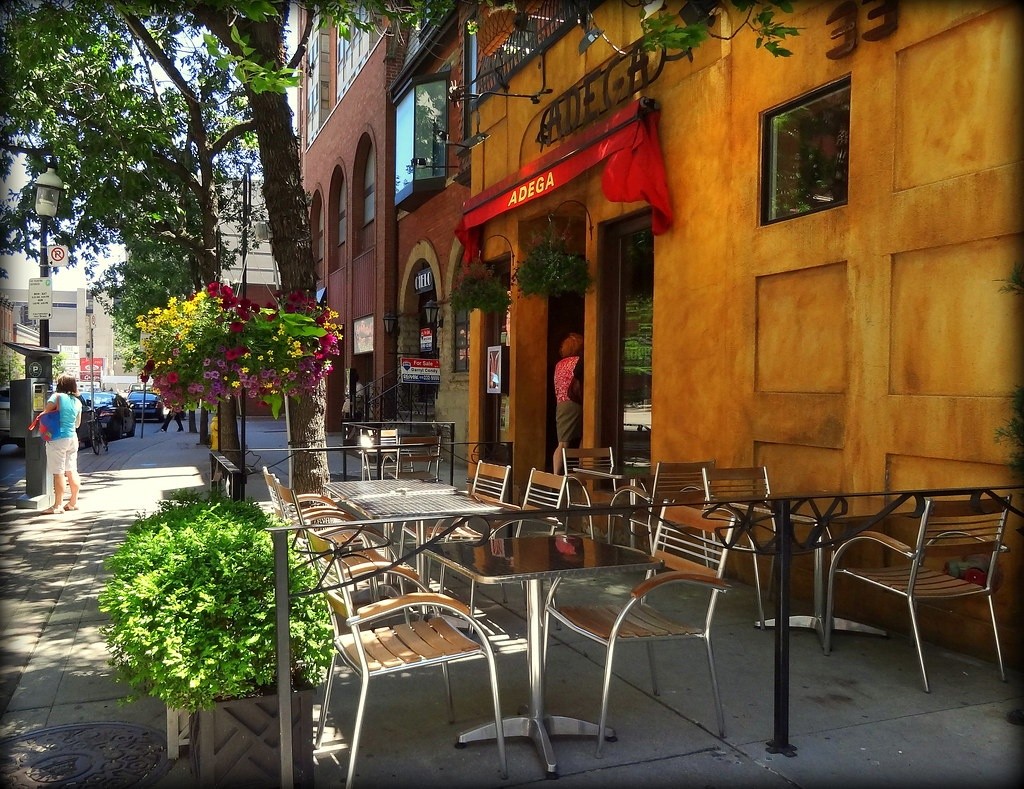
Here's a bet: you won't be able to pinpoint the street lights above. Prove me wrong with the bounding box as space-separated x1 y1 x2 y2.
32 166 63 349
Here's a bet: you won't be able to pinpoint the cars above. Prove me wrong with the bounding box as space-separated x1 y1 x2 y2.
75 394 95 447
77 391 136 440
126 391 164 422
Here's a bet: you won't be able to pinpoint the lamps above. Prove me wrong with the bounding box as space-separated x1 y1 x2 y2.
679 0 724 26
437 129 468 149
412 157 459 169
464 92 540 149
578 28 641 56
383 311 401 339
423 300 444 330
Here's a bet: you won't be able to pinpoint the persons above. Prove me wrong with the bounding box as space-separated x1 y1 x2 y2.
38 374 83 515
553 333 584 477
101 388 114 391
209 413 218 492
160 403 184 432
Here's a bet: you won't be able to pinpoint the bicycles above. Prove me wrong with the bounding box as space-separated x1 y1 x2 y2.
85 405 109 455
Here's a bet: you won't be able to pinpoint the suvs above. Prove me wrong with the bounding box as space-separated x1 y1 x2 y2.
0 386 25 447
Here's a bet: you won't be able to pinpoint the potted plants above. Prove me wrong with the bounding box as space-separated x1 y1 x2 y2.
98 486 339 788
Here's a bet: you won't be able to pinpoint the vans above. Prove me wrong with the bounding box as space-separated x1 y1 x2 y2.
128 383 153 393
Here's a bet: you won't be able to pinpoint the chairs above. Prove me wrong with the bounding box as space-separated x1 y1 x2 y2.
262 429 1011 789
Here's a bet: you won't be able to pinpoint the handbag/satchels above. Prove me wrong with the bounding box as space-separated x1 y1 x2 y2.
29 393 61 442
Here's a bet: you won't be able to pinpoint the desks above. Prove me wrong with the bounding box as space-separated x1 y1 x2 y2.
417 535 665 782
572 467 656 547
731 489 928 655
346 492 504 635
322 480 459 614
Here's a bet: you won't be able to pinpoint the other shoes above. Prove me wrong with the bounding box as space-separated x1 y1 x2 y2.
41 506 65 515
175 429 184 433
63 502 79 511
153 428 167 434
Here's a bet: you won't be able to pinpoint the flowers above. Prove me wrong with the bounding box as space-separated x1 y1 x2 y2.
126 281 345 417
449 257 513 315
510 229 594 297
